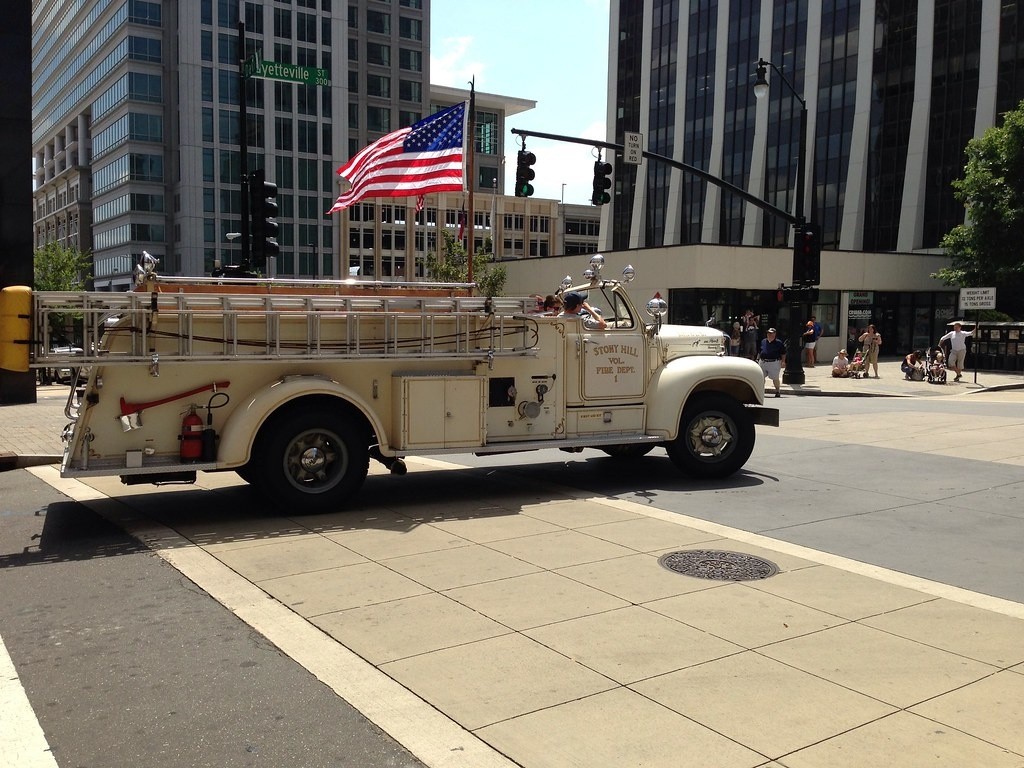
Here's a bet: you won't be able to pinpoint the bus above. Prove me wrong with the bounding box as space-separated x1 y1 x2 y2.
946 318 1024 370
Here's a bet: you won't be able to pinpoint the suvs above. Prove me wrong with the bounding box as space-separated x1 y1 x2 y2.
44 346 84 384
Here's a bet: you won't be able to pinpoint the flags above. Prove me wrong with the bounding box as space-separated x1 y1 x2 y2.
326 101 466 216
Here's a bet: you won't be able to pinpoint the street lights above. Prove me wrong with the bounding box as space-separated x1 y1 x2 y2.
754 57 807 384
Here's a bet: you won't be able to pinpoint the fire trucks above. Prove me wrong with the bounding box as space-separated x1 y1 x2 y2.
1 251 781 518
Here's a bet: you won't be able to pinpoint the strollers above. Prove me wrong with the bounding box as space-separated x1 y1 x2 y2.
847 348 870 379
926 344 946 384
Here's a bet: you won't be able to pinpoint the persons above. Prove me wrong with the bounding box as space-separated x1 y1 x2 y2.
730 309 759 361
754 327 787 398
930 352 944 379
559 292 607 329
527 294 543 314
541 294 563 317
850 325 882 378
802 316 823 367
832 349 850 377
900 350 925 379
939 323 978 381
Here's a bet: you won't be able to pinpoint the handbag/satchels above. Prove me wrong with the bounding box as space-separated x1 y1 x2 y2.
911 368 924 381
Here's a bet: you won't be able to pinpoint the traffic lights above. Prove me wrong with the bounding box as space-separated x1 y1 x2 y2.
592 160 613 205
776 288 788 303
248 169 280 267
515 149 537 197
801 224 821 287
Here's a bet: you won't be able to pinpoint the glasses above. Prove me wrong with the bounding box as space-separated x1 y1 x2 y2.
548 306 560 311
538 302 544 305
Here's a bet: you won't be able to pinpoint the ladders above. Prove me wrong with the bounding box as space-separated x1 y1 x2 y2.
1 286 543 372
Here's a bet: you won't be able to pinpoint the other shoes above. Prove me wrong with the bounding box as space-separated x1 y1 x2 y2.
804 364 814 368
775 392 781 397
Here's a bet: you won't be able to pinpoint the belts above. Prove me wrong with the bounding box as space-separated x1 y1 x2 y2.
763 359 780 362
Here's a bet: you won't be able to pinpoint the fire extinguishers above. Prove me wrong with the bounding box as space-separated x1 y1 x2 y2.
180 402 203 463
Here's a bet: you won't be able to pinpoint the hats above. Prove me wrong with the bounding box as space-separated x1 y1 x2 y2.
806 321 813 326
766 327 776 333
563 291 589 308
836 348 849 357
